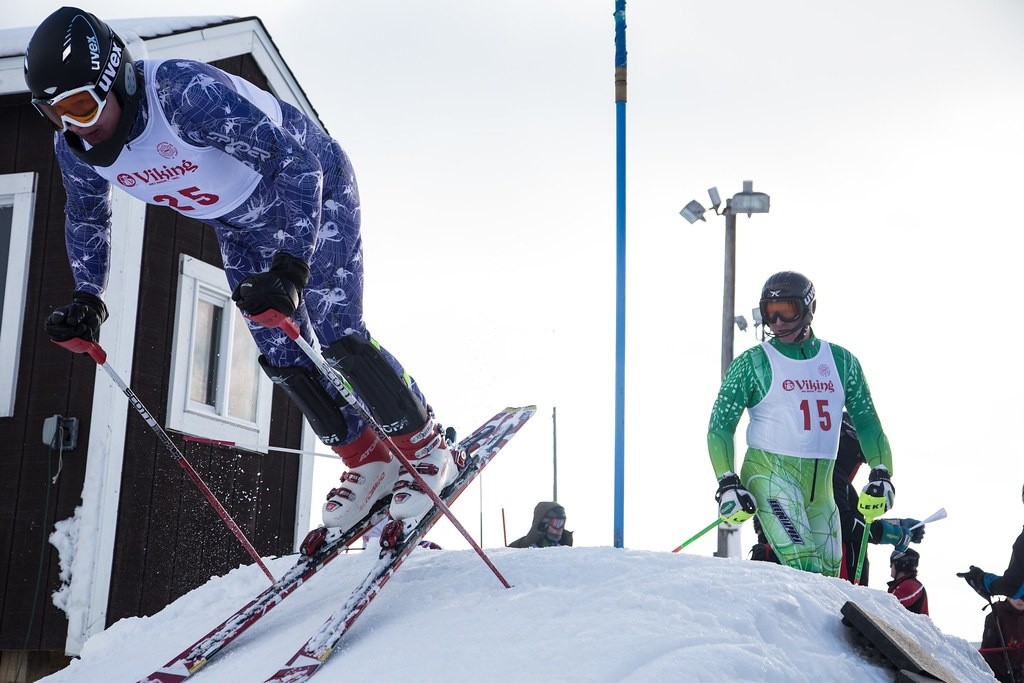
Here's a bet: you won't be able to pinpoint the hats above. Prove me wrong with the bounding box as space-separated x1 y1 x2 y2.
890 547 920 574
760 270 816 317
24 7 136 102
538 507 566 532
754 516 767 543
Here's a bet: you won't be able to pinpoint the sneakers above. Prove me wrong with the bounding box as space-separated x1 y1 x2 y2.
299 427 402 528
379 415 467 520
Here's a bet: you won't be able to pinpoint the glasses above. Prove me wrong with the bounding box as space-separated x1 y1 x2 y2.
761 299 803 323
31 85 106 133
550 518 566 529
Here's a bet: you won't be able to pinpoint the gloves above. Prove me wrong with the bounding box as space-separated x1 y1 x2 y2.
230 252 311 317
880 519 914 552
957 565 996 596
44 291 109 344
856 470 895 517
716 476 758 524
902 519 925 543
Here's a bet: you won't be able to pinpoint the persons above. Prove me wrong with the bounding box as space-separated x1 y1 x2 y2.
362 517 441 550
957 525 1024 600
708 270 897 578
981 596 1024 683
508 502 573 548
887 548 929 616
753 412 925 586
24 6 460 522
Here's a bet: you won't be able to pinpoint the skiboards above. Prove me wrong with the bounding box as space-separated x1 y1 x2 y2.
133 404 537 683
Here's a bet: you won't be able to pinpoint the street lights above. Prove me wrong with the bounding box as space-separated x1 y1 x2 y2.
679 180 770 559
